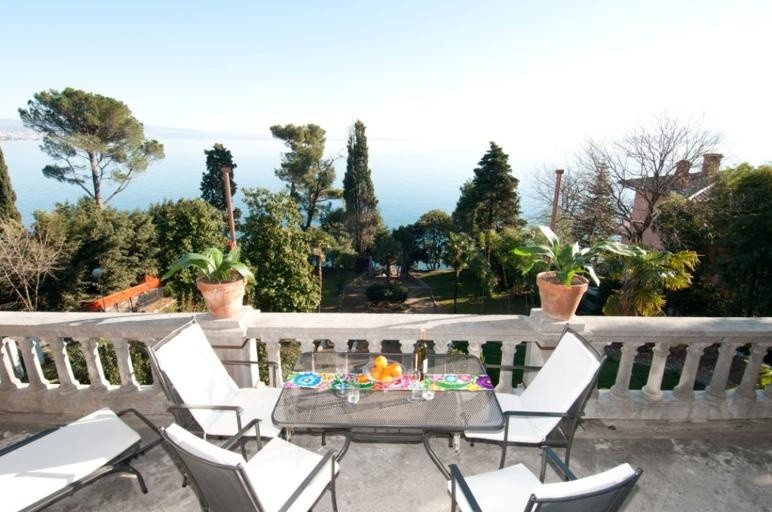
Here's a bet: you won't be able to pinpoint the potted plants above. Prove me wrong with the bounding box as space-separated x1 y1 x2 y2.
159 242 258 319
508 222 646 321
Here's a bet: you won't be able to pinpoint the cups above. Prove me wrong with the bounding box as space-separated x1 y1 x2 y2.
346 377 360 404
420 377 435 401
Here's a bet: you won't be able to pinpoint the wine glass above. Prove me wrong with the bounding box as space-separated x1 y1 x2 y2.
406 368 422 402
335 366 348 398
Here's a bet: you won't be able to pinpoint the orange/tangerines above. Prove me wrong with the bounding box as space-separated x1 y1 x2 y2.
357 375 368 383
372 356 401 380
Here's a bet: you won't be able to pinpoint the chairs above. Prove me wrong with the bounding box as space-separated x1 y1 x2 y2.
464 322 608 469
0 407 164 512
145 314 294 462
447 443 643 512
158 418 341 512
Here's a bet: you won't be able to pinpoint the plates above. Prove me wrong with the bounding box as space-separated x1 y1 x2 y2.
364 359 405 382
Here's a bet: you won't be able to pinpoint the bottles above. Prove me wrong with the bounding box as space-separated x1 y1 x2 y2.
412 327 430 373
